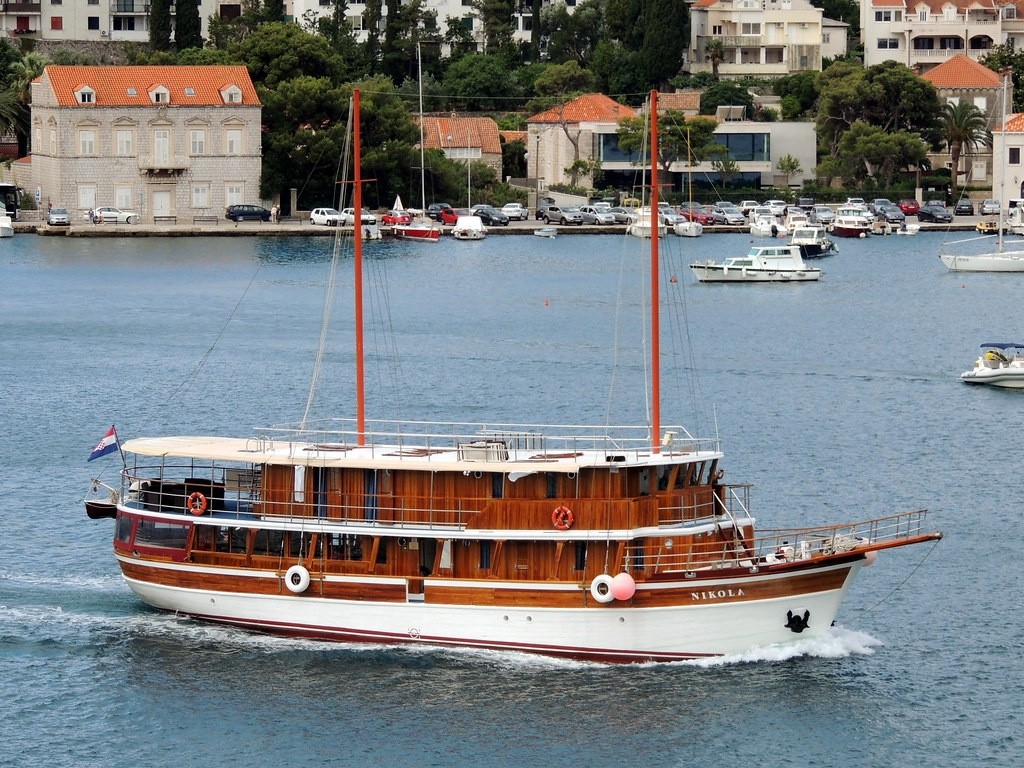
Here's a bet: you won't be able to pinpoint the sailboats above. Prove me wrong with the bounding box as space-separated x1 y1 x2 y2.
451 123 488 239
938 75 1024 272
625 96 667 239
673 129 703 237
391 43 439 241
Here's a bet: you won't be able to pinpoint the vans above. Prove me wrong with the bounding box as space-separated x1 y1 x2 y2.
225 205 277 222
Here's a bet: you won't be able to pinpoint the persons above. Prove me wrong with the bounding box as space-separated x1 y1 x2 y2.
707 467 724 486
659 469 672 490
88 208 94 227
270 205 280 223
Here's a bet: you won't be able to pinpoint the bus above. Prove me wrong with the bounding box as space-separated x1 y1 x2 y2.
0 184 25 222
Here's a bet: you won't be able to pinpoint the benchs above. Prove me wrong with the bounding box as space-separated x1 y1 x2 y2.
93 217 118 225
153 216 177 225
277 216 303 226
236 216 261 226
193 216 218 226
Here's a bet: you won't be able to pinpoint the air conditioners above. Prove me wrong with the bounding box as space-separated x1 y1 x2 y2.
801 23 808 29
775 23 783 28
101 30 109 37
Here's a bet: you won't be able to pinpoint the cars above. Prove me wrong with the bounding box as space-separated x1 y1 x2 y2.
381 211 412 226
46 208 71 226
83 206 141 224
310 208 346 226
341 208 377 226
426 198 1001 228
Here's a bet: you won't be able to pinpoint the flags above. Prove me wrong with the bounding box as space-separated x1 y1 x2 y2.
88 427 118 462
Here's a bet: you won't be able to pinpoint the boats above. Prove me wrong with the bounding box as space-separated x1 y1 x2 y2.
960 343 1024 388
749 207 892 238
114 88 944 665
786 223 839 259
977 221 1008 235
534 228 558 236
1005 202 1024 234
897 222 920 236
688 246 821 282
84 424 132 519
361 225 382 239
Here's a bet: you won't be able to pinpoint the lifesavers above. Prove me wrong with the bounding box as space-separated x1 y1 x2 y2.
985 350 1006 361
552 506 574 531
590 574 615 603
284 565 312 593
187 492 207 516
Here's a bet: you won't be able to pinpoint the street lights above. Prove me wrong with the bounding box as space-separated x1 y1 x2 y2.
536 136 542 210
